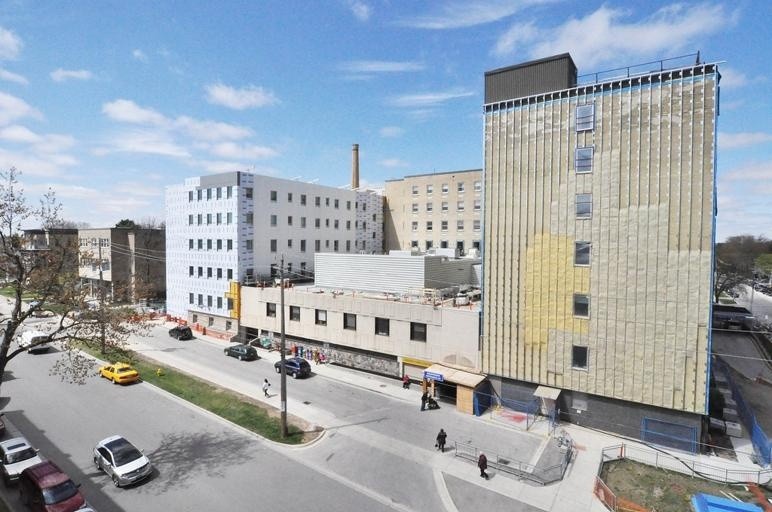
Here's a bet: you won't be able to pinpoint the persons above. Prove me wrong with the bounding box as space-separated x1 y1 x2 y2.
402 373 410 391
320 352 325 364
436 428 447 453
476 450 489 480
420 390 430 411
313 349 320 365
262 378 271 398
427 393 440 409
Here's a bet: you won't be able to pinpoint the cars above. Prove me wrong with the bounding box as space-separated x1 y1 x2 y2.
19 460 88 512
274 358 311 379
74 312 86 321
93 434 153 488
28 304 48 318
169 326 192 340
99 362 140 384
0 436 44 486
224 344 257 360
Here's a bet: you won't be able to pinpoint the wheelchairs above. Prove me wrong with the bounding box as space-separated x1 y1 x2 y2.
426 400 439 409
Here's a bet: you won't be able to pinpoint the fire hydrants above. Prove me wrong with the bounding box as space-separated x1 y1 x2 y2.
156 368 162 376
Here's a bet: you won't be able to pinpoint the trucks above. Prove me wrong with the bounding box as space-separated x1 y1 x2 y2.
16 331 50 354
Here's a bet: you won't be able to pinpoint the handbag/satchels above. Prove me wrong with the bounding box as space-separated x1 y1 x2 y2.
435 443 439 447
268 383 271 386
